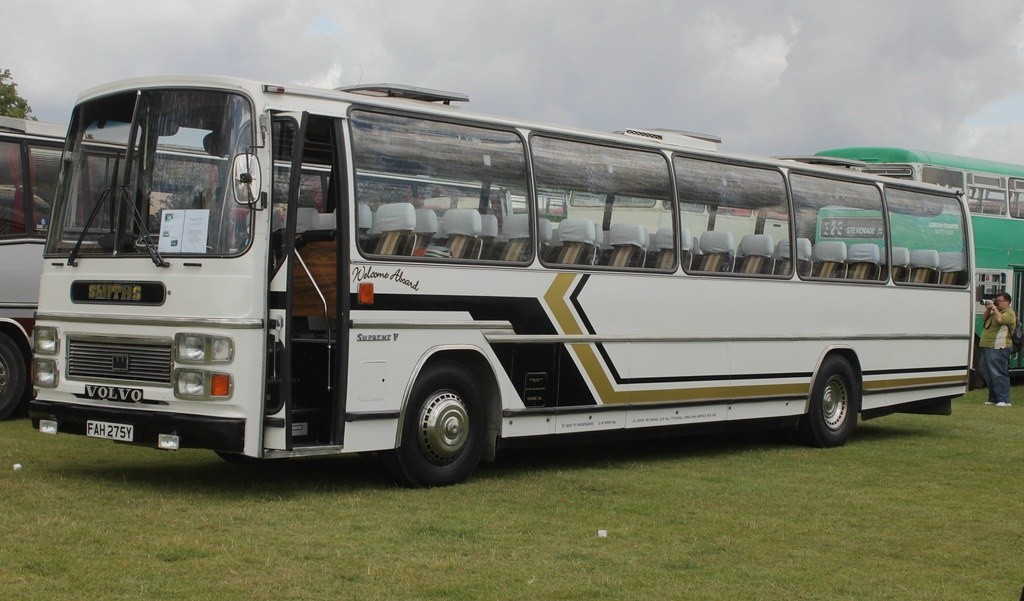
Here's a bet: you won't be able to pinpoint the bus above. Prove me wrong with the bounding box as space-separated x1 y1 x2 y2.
0 114 514 420
24 71 979 490
799 146 1024 389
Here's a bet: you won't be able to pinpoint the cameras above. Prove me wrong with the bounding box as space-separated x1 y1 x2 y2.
980 299 998 306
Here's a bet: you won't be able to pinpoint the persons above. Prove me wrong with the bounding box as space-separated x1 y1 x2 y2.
979 292 1016 406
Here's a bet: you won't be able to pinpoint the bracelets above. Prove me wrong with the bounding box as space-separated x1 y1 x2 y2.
992 306 996 309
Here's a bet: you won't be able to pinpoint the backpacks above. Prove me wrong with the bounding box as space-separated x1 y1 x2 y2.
1002 311 1022 353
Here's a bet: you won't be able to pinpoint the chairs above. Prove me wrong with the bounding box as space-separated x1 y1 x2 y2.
247 202 968 285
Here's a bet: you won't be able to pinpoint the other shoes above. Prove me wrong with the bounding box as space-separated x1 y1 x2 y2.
984 401 995 405
996 401 1012 406
